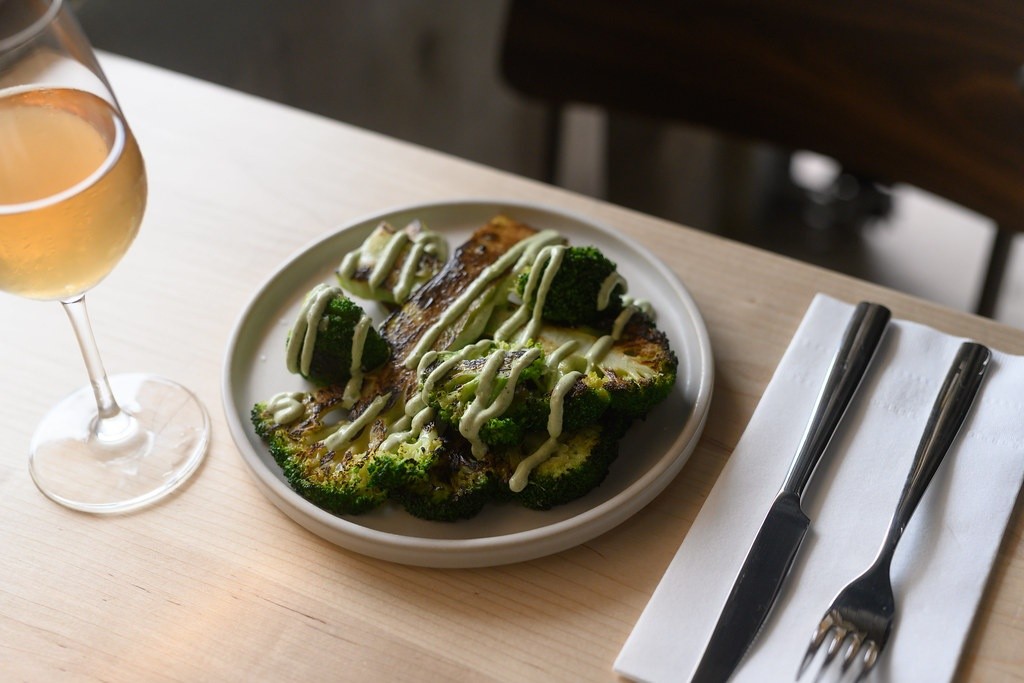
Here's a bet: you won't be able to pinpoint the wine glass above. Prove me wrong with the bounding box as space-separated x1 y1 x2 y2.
0 0 210 516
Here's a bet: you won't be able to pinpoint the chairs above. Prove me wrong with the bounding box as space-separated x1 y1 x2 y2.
499 0 1023 318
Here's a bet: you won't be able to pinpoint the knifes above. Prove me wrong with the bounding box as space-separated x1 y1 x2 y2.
689 300 892 683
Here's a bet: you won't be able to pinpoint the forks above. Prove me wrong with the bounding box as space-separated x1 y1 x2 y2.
795 342 992 683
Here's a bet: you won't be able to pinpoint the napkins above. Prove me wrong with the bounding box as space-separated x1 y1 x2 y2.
613 293 1024 683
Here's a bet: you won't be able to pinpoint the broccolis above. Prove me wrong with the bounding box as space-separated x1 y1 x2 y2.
250 215 680 522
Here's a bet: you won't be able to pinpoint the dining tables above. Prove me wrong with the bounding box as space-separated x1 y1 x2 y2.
0 45 1024 683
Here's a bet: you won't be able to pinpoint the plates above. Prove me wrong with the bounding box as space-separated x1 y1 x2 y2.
221 200 715 569
